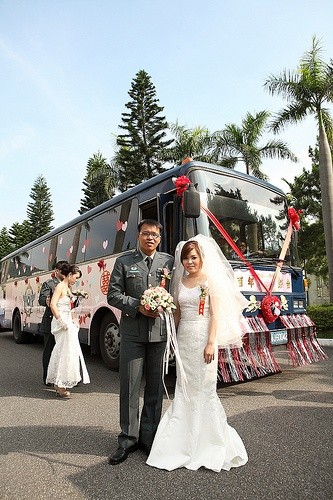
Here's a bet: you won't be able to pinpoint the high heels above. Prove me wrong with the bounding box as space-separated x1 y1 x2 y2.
54 384 70 398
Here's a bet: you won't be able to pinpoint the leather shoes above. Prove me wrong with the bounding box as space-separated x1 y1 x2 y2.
137 440 151 457
109 444 138 465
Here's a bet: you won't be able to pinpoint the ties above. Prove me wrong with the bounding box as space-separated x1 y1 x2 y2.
144 256 153 271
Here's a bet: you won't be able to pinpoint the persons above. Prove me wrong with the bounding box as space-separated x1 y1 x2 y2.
38 261 72 386
146 233 250 474
230 238 248 259
106 220 174 465
49 264 90 398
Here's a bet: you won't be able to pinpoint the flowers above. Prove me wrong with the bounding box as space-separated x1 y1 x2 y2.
280 295 289 312
141 287 177 316
271 301 281 316
72 288 88 307
247 296 261 313
161 265 172 279
199 283 209 299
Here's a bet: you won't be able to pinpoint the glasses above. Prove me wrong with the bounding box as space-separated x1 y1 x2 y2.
139 233 162 239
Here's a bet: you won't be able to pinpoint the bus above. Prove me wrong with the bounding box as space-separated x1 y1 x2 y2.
0 157 330 385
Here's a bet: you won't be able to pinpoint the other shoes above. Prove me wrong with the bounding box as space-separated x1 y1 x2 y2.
43 380 52 387
